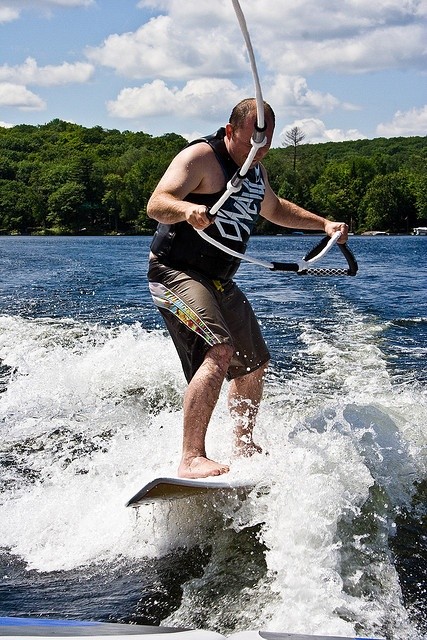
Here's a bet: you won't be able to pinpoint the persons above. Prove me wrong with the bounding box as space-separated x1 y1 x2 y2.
143 97 349 481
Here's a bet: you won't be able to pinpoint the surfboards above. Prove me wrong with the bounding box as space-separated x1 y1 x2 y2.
125 477 258 508
